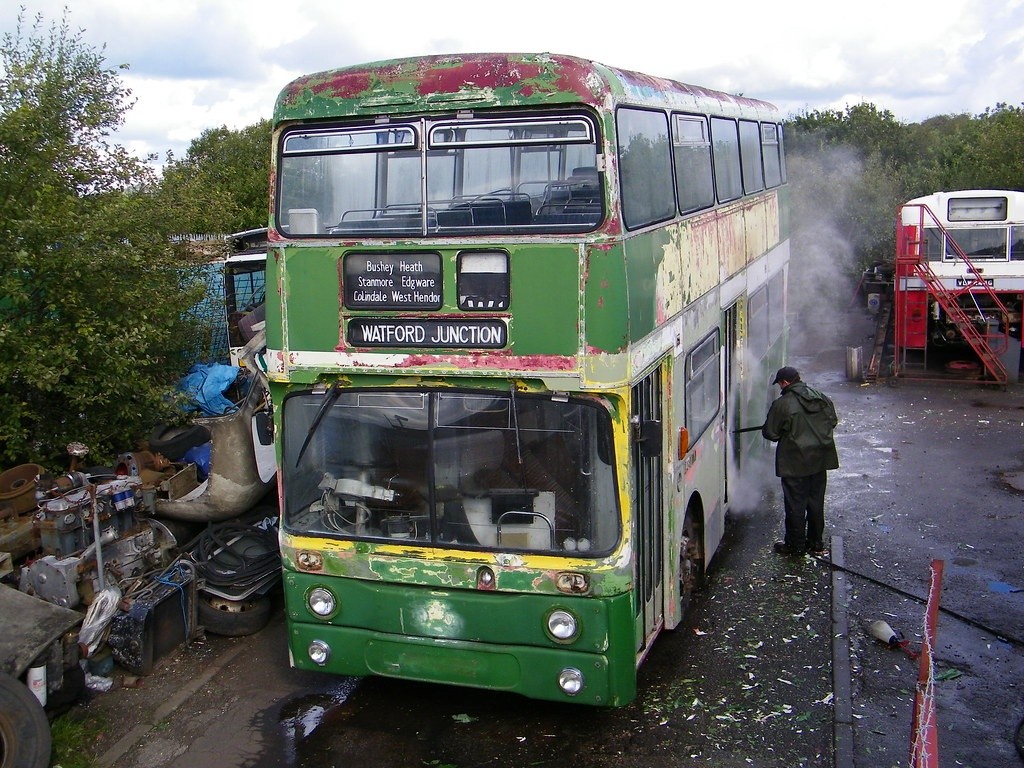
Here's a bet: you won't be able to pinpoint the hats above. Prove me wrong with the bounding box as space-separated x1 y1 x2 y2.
772 367 797 386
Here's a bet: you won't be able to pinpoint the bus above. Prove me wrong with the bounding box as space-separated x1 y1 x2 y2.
263 51 796 710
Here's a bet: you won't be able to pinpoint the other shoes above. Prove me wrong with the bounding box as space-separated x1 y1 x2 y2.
806 539 822 552
774 541 805 555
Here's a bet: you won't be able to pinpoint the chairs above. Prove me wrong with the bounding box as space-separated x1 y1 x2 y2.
330 166 602 234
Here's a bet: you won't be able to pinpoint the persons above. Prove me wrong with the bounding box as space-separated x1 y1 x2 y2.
762 366 840 557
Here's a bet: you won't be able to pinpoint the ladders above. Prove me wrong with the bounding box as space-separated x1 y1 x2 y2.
902 234 930 371
